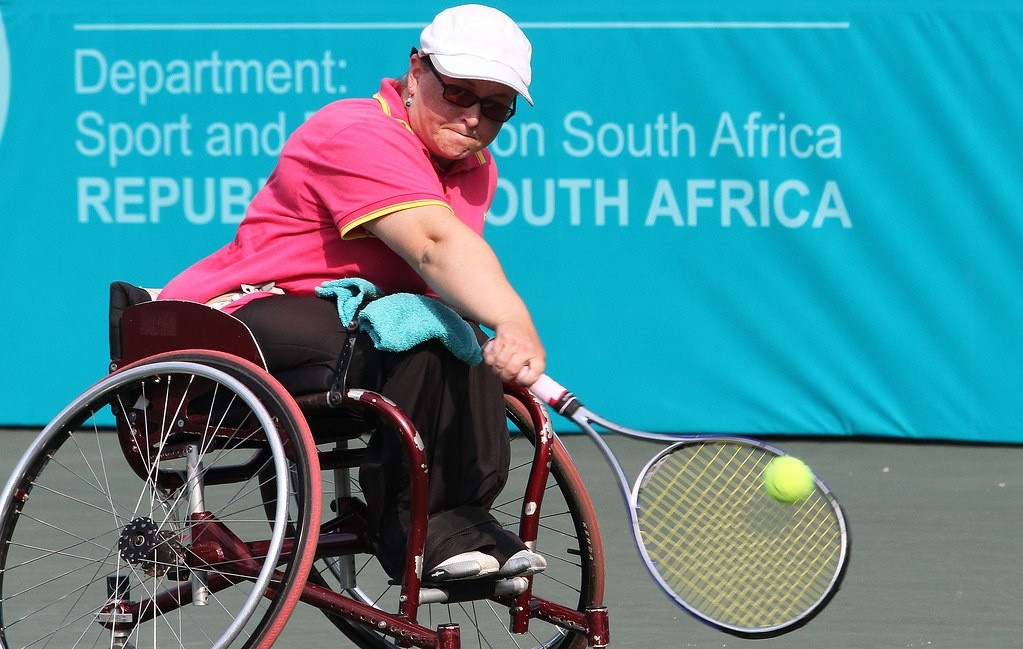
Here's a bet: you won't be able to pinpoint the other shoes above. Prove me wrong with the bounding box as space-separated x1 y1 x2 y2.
498 548 546 576
426 551 498 581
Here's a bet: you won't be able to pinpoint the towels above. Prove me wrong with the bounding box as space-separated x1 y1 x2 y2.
314 277 484 369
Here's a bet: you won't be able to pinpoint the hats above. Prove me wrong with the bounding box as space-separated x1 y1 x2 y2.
418 4 535 107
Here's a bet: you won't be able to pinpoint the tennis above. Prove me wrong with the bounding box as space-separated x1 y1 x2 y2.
764 456 815 506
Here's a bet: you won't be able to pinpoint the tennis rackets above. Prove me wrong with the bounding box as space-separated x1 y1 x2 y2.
480 339 852 640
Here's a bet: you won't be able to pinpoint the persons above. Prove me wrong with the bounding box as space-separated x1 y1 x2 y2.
154 3 551 591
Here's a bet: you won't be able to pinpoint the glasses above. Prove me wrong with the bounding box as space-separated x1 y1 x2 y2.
423 54 517 124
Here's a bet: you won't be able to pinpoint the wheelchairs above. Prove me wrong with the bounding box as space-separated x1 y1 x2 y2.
0 282 610 648
